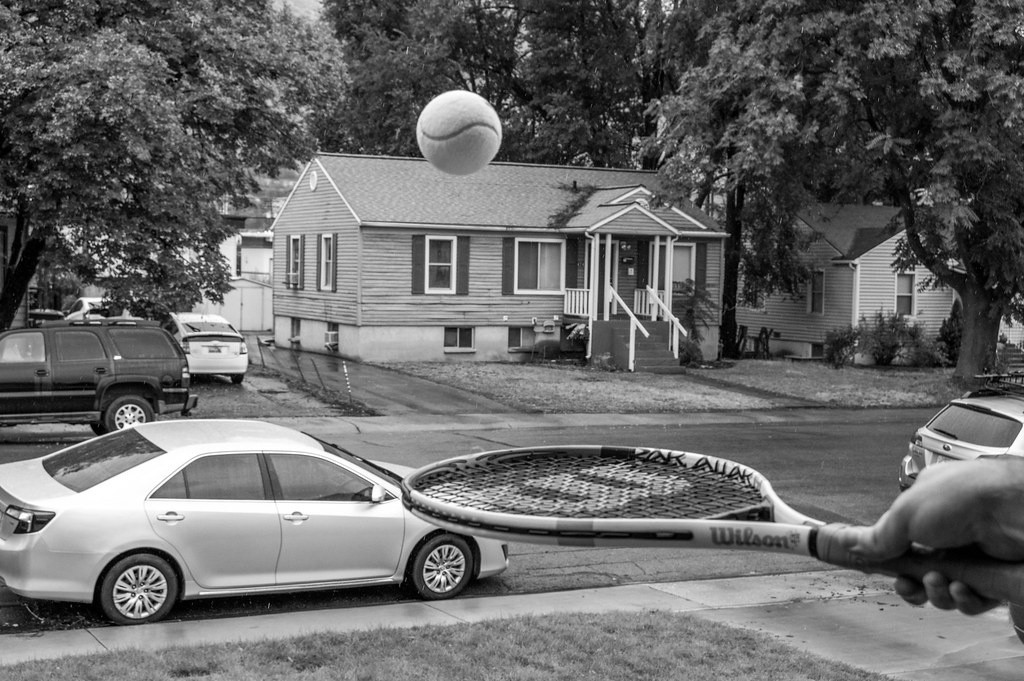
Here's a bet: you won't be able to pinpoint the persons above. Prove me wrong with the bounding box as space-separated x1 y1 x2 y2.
842 459 1023 614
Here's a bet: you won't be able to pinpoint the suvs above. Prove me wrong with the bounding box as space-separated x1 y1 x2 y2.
0 319 200 434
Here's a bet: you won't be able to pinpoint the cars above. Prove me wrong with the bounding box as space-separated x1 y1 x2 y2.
64 297 136 328
1 418 512 623
161 311 249 384
896 369 1023 490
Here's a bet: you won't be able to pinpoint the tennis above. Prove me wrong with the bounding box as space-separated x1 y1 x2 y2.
417 88 504 175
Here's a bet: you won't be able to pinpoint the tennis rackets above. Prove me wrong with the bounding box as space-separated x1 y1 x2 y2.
400 443 1023 605
